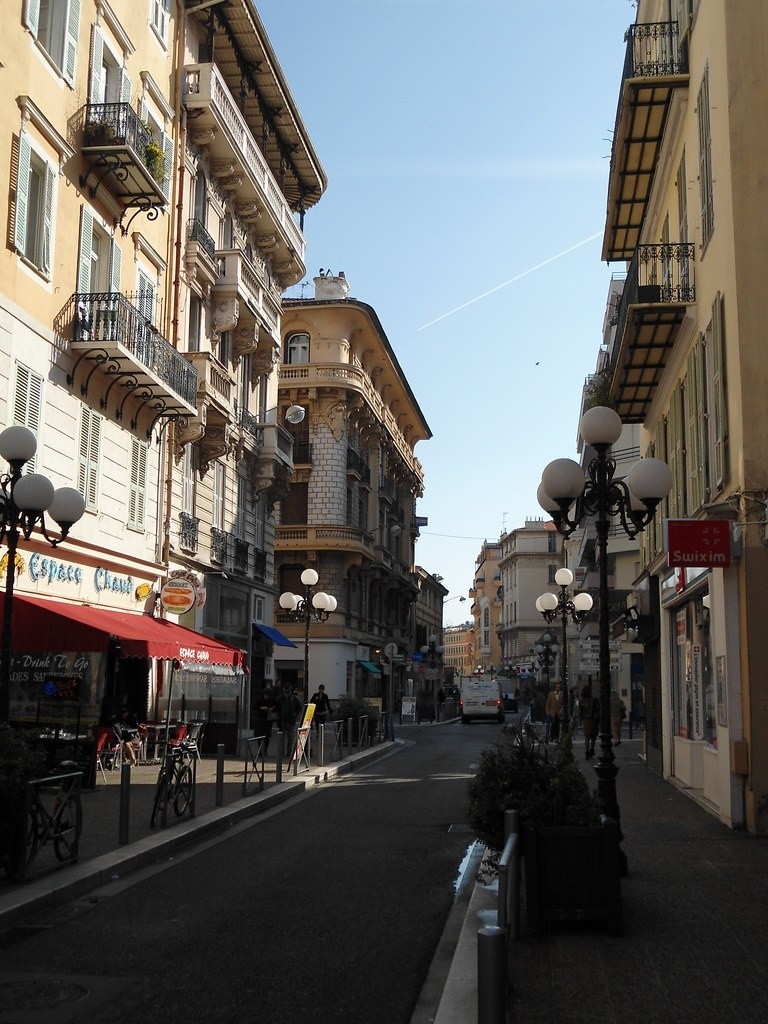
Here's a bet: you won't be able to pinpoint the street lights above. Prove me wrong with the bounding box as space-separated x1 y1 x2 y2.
0 426 87 728
534 404 677 881
535 566 594 777
278 567 338 759
420 634 445 724
536 633 561 725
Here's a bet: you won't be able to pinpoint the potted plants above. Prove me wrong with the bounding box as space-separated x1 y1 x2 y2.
463 685 600 887
332 693 383 745
89 118 166 186
403 687 436 726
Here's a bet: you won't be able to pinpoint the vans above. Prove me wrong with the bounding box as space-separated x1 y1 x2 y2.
460 680 509 724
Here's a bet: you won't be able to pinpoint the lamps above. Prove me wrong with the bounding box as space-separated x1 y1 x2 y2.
369 525 401 536
239 402 305 424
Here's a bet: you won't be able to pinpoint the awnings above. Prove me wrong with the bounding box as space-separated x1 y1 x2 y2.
360 660 381 673
0 591 242 667
255 624 298 648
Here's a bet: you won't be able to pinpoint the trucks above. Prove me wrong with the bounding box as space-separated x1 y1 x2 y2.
492 679 521 713
452 675 480 696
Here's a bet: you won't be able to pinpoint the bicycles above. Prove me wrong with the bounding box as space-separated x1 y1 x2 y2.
149 737 199 828
0 761 85 888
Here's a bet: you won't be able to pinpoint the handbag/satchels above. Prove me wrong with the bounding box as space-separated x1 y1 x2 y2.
583 715 598 736
622 711 626 719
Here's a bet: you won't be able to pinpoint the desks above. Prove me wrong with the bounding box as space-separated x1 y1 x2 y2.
121 729 138 733
147 725 176 762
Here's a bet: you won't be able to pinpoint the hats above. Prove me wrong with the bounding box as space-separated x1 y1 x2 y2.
282 682 292 691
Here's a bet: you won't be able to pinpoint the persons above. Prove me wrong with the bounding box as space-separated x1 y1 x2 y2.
546 682 564 742
277 684 303 756
436 688 445 711
578 686 601 759
454 687 460 701
611 690 625 746
310 684 333 739
110 702 140 765
251 690 277 757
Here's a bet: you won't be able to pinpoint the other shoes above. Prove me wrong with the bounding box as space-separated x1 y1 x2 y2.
615 741 621 746
586 751 589 759
590 749 595 756
106 761 112 770
131 759 136 768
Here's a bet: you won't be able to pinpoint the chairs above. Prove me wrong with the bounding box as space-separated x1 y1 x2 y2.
96 719 204 771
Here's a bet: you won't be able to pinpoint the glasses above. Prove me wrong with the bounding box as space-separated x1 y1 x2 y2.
119 711 128 713
